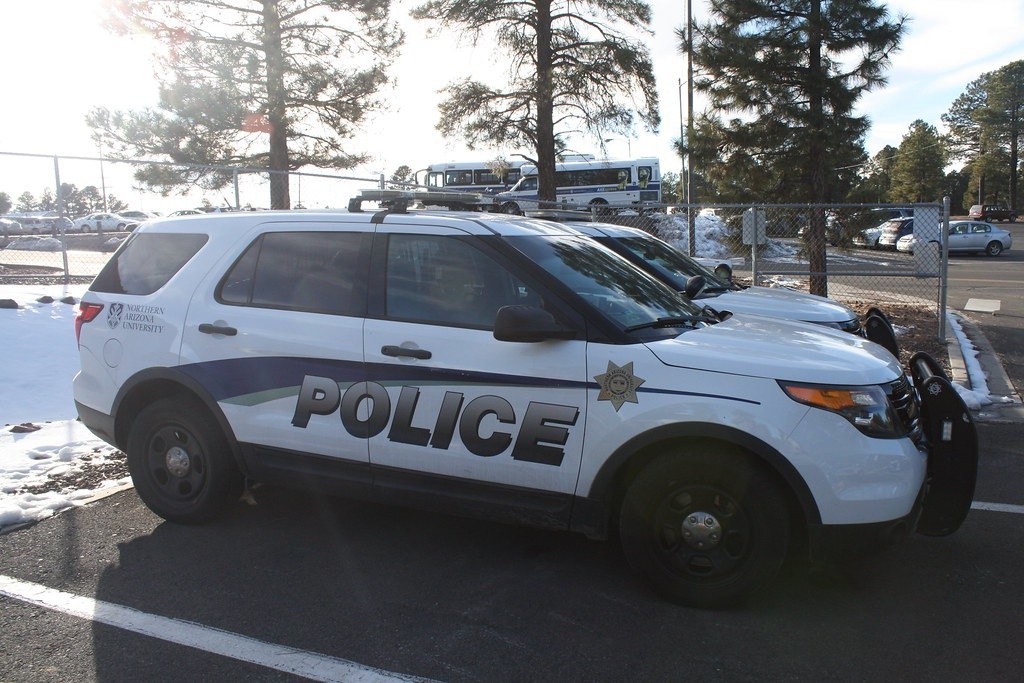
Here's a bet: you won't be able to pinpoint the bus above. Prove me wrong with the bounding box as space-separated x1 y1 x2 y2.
413 160 540 211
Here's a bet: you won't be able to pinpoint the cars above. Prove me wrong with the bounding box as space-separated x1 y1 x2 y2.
851 217 905 250
0 207 267 237
896 220 1012 258
797 209 839 242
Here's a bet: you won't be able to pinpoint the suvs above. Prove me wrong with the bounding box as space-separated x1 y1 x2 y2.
522 202 902 364
967 204 1019 223
878 219 914 252
72 188 977 610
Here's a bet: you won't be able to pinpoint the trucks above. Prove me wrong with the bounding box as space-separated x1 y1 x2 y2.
492 159 641 218
636 156 661 208
